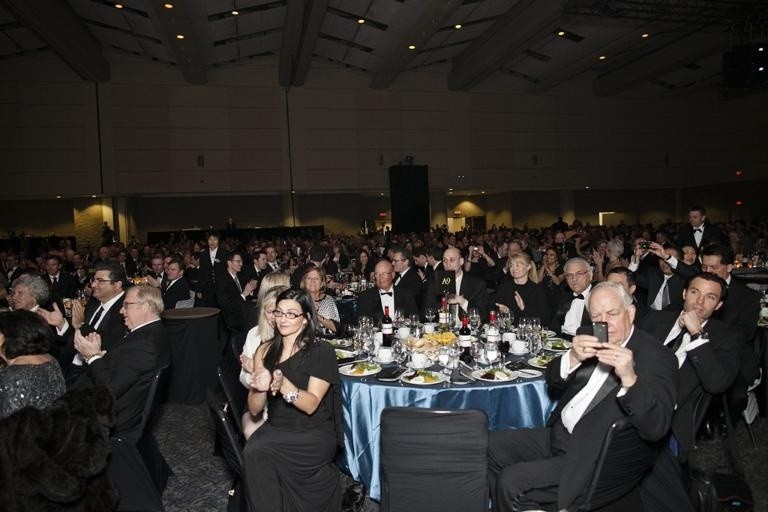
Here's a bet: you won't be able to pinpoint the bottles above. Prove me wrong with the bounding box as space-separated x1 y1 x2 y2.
759 246 765 269
590 258 595 280
751 247 758 267
741 249 748 268
381 307 392 346
438 299 451 323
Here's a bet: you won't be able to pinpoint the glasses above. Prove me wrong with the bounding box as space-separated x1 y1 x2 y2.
123 301 142 309
272 310 304 319
563 271 588 279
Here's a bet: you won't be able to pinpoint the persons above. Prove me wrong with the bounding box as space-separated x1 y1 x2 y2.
1 208 768 512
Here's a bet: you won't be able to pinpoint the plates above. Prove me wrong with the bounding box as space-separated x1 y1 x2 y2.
334 348 356 359
543 330 557 338
472 369 518 381
555 351 565 357
517 370 542 378
542 338 571 352
400 371 447 385
526 355 562 369
339 364 382 377
336 339 352 348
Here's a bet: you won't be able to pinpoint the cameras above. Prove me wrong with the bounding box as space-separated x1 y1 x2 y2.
473 247 479 251
639 242 651 249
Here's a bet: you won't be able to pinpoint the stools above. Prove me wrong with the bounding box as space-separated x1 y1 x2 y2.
701 379 763 448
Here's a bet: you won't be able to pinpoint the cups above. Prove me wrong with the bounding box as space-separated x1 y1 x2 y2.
335 271 368 297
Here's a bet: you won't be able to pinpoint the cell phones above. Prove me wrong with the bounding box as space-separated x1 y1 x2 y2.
592 322 608 343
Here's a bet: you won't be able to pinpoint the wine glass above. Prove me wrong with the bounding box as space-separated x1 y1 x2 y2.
343 309 542 370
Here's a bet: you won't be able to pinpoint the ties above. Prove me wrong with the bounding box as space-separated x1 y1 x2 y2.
662 281 668 309
164 280 171 291
90 306 104 327
665 327 688 353
52 277 57 290
235 277 241 293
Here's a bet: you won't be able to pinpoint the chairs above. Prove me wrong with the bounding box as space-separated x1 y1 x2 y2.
688 390 712 444
579 415 654 509
379 406 490 512
108 358 177 509
207 332 366 511
1 394 112 511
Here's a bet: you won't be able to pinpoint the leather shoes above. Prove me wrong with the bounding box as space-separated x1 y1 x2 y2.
698 411 730 441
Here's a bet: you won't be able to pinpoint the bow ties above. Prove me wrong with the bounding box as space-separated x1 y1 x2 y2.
570 294 584 299
381 292 392 296
693 229 702 233
396 273 401 278
154 273 162 279
